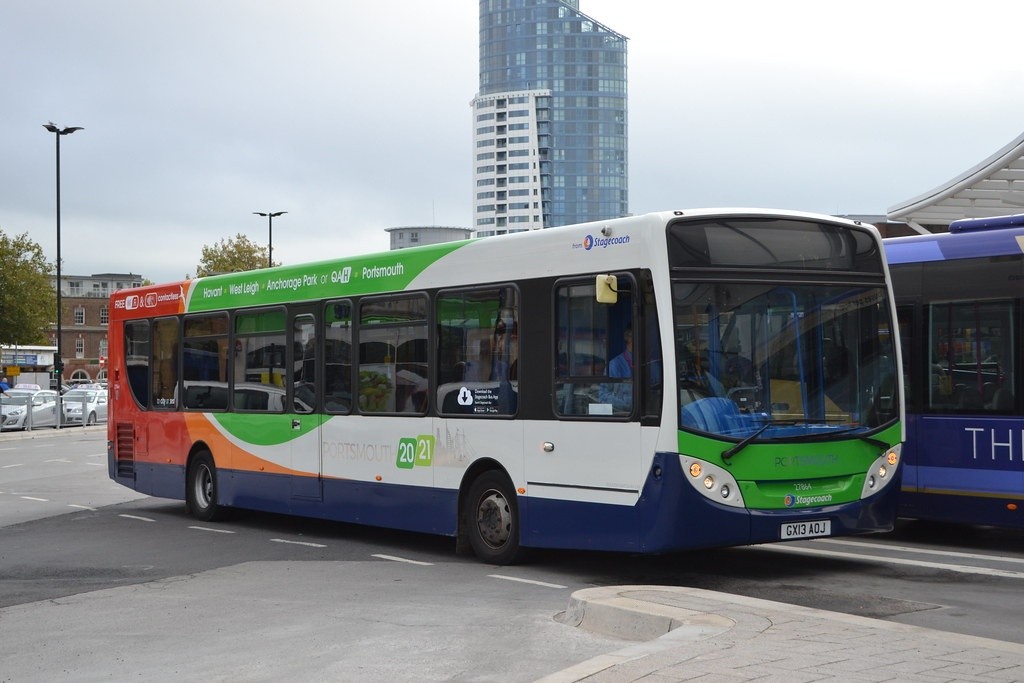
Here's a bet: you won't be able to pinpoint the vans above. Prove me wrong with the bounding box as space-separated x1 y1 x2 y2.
173 377 313 413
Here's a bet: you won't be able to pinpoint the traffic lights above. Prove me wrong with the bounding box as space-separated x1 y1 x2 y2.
54 353 65 374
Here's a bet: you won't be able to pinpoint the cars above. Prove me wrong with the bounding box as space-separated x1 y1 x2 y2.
0 383 108 432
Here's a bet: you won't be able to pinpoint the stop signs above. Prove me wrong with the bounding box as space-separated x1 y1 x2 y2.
99 356 105 368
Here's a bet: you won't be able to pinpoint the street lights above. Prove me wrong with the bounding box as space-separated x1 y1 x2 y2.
252 211 289 268
42 121 86 396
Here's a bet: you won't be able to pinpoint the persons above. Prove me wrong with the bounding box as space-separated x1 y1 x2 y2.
1 378 9 391
599 325 662 413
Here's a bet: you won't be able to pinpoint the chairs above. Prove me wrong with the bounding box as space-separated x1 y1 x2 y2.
246 338 459 411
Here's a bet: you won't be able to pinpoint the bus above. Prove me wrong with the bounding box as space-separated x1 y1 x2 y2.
106 208 1024 564
